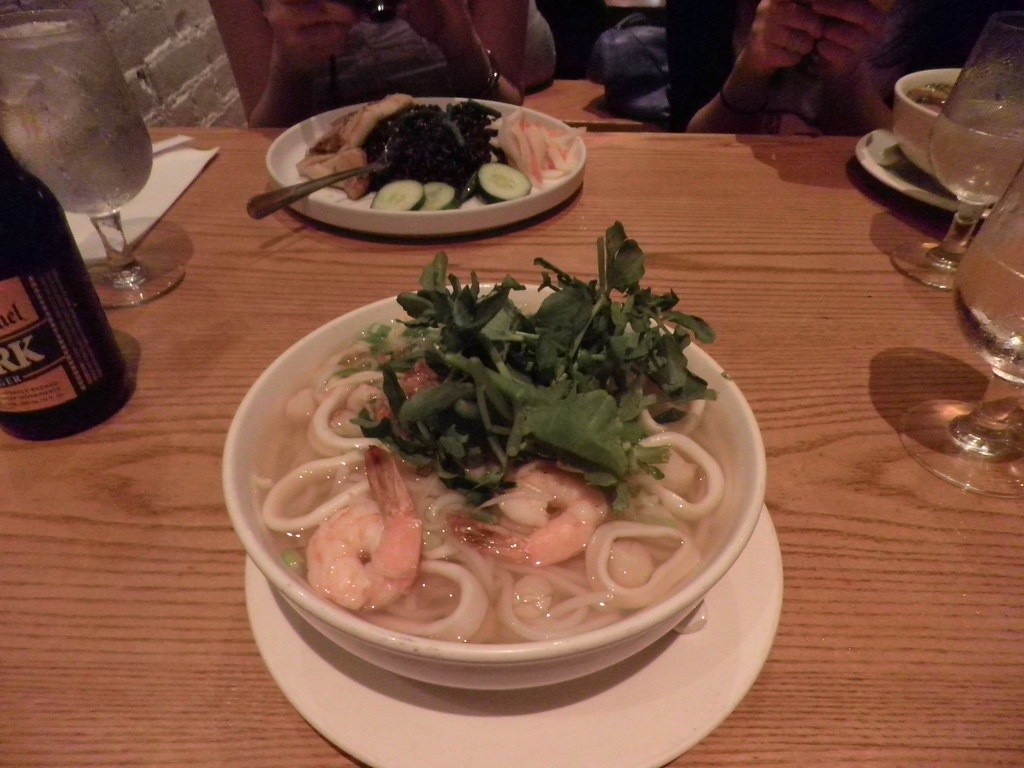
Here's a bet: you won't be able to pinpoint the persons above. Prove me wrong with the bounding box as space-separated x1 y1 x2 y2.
665 0 991 136
210 1 554 129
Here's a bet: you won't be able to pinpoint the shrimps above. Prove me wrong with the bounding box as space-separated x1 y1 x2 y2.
301 443 615 619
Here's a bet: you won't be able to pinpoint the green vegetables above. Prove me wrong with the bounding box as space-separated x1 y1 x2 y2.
364 98 505 200
331 219 719 525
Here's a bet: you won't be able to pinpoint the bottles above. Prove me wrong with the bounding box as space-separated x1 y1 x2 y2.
0 137 126 441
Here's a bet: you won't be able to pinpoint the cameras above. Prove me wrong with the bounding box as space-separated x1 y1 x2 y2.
339 0 400 24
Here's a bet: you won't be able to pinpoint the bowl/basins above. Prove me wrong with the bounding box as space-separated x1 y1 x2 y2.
220 284 767 691
892 67 964 176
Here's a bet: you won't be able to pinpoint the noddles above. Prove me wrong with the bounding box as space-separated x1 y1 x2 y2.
259 337 728 645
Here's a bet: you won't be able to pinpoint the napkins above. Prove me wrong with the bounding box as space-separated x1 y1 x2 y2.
64 133 220 270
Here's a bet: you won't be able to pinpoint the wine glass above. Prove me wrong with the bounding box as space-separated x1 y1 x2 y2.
902 160 1024 497
890 14 1024 291
0 9 184 308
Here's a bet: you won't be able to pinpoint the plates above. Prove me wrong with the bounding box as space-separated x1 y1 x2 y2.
245 502 785 768
266 97 585 235
855 128 997 218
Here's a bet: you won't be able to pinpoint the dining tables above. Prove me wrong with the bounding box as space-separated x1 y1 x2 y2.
0 127 1024 768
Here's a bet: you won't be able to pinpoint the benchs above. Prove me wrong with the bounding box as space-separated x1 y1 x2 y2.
521 78 670 133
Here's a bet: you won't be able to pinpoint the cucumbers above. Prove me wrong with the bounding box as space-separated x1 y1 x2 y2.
369 179 426 210
477 162 532 204
415 180 459 211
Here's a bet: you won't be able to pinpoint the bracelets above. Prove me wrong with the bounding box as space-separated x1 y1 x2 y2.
718 90 768 116
478 48 502 100
